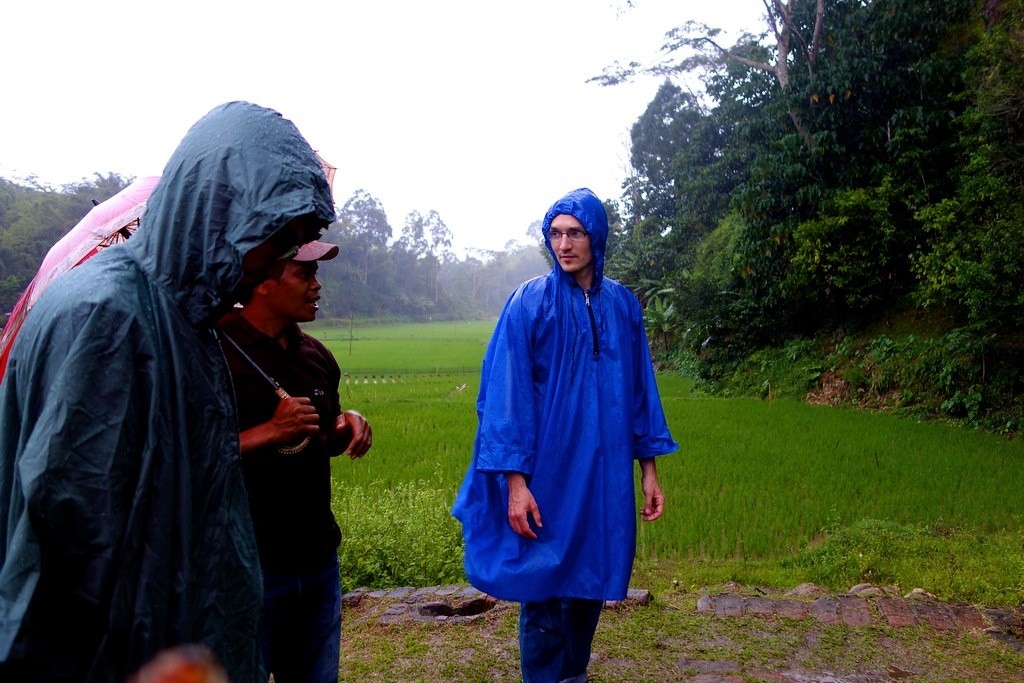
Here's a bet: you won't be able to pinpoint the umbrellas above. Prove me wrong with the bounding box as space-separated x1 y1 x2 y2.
0 177 314 454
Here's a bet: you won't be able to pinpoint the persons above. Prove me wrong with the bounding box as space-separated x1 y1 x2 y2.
0 100 337 683
214 241 373 683
449 189 680 683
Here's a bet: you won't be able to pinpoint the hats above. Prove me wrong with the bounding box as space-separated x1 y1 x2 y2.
292 239 340 261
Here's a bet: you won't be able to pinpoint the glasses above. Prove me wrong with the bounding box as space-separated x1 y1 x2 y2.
271 224 298 260
547 230 588 241
